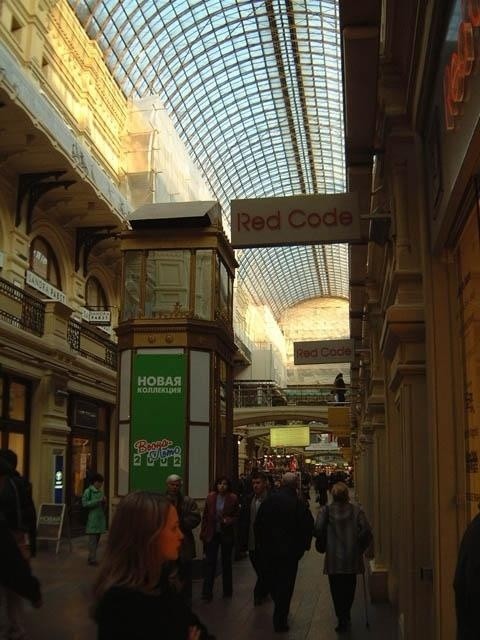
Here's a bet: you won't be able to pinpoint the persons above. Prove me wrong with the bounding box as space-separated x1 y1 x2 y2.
314 470 355 509
82 467 107 564
335 373 346 406
331 390 339 403
0 463 46 609
453 507 480 639
87 490 218 640
160 465 314 632
314 483 372 634
1 448 40 639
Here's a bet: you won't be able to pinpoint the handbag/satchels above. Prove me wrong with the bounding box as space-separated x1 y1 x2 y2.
315 537 327 553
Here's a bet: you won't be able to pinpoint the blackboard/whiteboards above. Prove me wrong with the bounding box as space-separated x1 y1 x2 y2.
34 503 66 541
